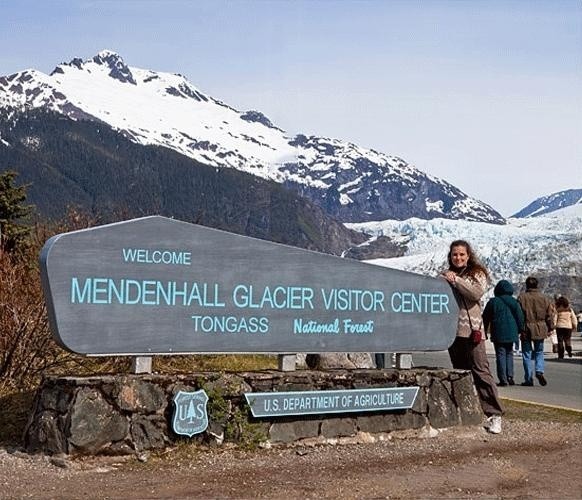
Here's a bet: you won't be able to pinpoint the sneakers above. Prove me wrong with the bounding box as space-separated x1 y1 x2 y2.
521 380 533 387
489 415 501 434
536 372 547 386
497 377 514 386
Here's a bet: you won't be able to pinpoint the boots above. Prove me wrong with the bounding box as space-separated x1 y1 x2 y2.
566 346 573 357
558 347 564 358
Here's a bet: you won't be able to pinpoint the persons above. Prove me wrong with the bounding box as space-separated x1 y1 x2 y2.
555 297 577 360
435 239 504 434
517 276 555 387
482 279 525 387
576 311 581 333
549 293 564 353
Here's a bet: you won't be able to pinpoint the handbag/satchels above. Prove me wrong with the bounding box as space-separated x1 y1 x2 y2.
470 330 482 345
